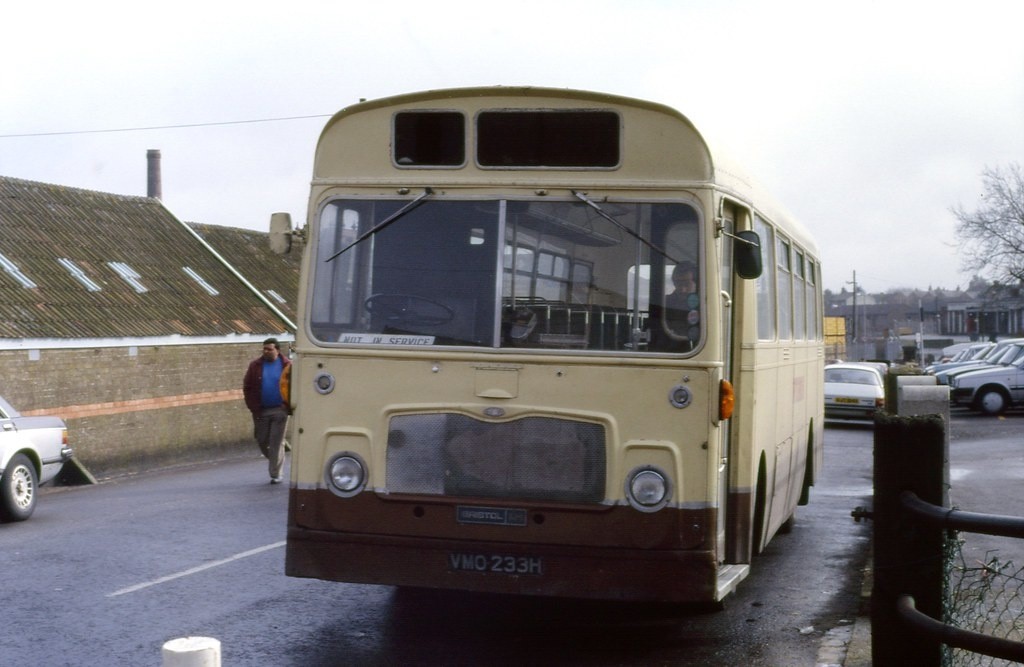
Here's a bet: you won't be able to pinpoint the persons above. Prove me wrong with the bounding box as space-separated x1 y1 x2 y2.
243 338 291 484
657 265 698 351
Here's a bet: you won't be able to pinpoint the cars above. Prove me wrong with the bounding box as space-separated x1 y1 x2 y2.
829 359 889 375
923 338 1024 416
0 395 73 520
824 364 885 420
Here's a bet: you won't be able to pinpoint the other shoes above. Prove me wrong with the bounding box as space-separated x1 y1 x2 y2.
271 476 282 483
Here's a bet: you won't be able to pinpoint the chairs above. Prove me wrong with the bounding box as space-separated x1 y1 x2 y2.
828 374 842 382
856 377 871 384
387 269 497 340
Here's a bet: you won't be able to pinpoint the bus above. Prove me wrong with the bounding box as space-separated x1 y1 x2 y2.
269 85 826 609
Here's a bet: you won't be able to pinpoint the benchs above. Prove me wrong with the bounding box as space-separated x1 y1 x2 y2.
501 295 648 352
